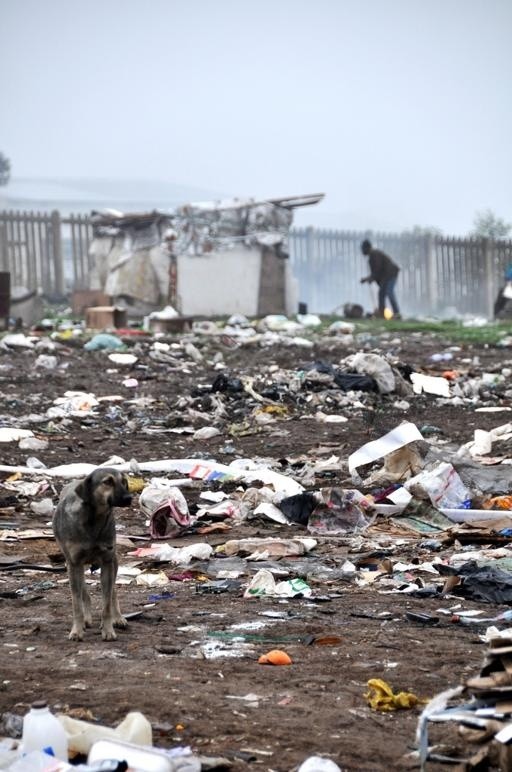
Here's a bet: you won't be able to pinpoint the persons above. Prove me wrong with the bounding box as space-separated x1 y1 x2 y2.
360 240 402 322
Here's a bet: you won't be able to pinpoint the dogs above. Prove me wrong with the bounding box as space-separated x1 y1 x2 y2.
52 468 133 642
